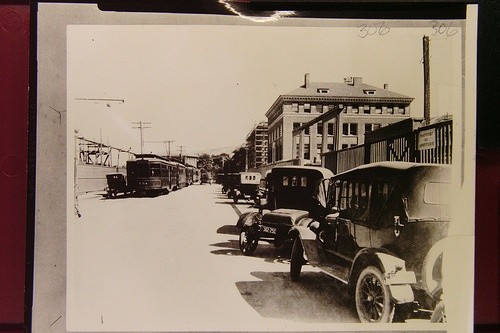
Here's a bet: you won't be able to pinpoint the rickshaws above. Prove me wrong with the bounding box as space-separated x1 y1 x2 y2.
104 174 127 199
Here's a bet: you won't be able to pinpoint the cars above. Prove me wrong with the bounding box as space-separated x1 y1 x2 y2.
222 172 263 204
236 165 334 254
286 161 447 325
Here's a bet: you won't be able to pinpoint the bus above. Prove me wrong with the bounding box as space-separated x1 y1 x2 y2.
126 153 201 197
